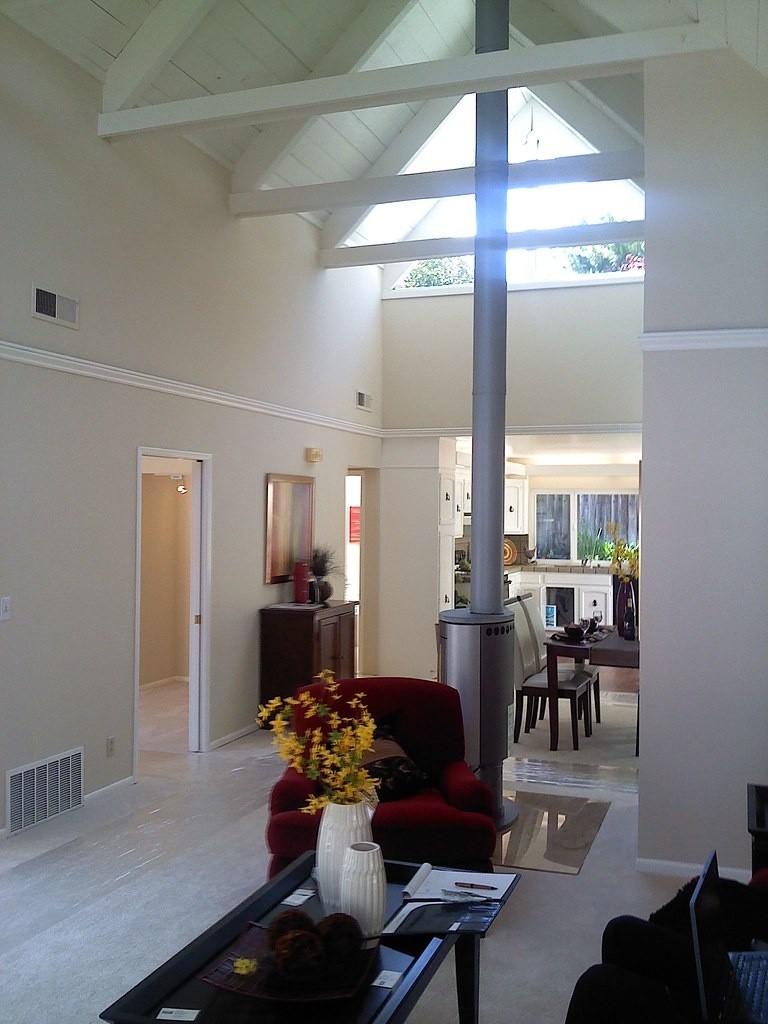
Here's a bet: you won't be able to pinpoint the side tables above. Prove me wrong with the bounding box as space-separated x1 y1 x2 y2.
747 783 768 876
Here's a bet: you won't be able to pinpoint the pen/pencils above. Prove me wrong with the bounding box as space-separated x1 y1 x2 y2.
454 882 498 890
441 888 493 900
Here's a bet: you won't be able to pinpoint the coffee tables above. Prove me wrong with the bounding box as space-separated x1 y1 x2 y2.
100 849 480 1024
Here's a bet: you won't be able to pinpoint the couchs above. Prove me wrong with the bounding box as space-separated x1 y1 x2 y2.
265 677 497 881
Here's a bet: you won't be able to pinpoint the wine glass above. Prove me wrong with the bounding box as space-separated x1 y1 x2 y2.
593 611 602 627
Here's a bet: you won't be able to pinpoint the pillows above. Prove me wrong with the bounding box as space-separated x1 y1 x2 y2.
326 711 431 802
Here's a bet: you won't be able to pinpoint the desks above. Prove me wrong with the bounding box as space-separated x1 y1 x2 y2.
543 626 639 756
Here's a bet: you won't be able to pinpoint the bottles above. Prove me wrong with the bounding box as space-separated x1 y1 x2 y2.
624 599 635 640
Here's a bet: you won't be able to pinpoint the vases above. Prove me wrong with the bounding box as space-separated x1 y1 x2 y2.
617 581 636 636
316 800 373 915
340 842 387 949
312 576 324 604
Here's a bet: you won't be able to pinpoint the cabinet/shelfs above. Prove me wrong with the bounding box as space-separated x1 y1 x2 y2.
504 479 528 535
580 585 613 626
260 600 360 722
380 469 472 682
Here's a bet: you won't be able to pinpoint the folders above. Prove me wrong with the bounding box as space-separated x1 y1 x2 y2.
382 867 522 936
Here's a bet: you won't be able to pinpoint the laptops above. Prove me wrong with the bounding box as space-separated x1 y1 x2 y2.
689 848 768 1024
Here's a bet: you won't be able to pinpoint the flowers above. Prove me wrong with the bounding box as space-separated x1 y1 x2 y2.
607 521 639 582
256 668 382 815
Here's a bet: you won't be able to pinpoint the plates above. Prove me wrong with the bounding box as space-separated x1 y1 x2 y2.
504 539 518 565
201 919 380 1001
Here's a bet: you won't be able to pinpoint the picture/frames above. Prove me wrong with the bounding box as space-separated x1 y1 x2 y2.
266 473 316 584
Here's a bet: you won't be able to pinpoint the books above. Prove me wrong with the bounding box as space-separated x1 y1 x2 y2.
382 863 517 934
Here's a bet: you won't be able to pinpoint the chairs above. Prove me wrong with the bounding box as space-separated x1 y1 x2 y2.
503 597 591 751
517 593 600 736
